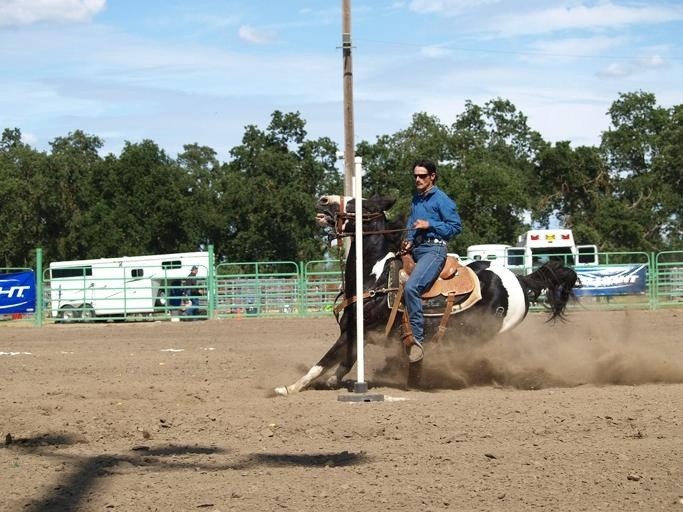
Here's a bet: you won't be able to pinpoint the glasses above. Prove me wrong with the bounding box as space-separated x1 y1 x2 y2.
412 173 431 178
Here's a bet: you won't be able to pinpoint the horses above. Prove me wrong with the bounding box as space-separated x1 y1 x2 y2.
273 195 587 397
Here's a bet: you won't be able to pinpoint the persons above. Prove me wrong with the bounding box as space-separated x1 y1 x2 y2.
396 159 463 365
180 265 207 322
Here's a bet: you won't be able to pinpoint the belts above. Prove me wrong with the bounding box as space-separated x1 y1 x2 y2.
416 234 447 245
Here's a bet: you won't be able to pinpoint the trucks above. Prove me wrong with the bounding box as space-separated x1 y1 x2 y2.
516 227 576 276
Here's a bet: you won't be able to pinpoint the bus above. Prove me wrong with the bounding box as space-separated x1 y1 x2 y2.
49 252 216 322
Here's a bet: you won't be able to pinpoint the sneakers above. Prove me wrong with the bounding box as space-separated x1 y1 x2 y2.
408 344 423 363
